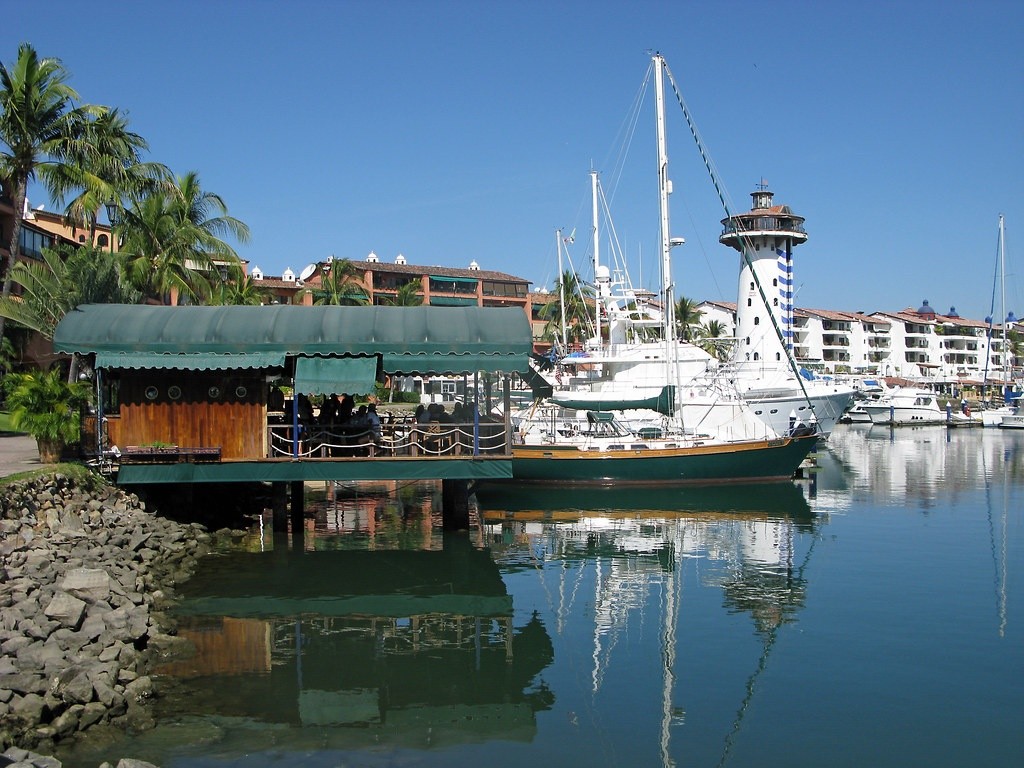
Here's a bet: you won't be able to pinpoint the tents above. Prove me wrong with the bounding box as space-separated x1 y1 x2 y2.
52 305 533 455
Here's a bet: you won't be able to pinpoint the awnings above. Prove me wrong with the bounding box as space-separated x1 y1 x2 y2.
374 294 424 299
430 297 478 305
314 292 368 300
429 276 479 282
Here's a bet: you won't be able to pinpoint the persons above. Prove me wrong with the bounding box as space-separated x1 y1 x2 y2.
415 401 481 452
272 386 381 455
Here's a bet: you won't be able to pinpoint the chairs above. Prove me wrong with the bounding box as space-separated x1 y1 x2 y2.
423 420 453 455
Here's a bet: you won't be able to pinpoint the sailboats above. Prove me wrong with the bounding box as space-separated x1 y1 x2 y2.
511 46 1024 487
